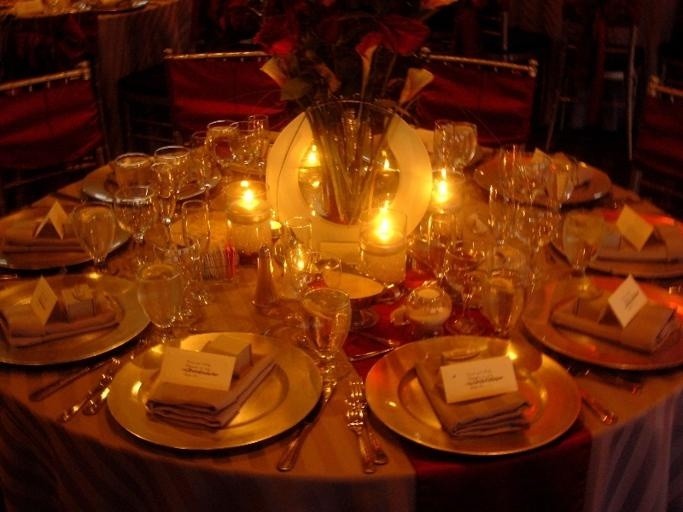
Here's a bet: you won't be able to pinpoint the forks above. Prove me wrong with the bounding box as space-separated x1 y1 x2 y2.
342 398 376 475
344 376 391 465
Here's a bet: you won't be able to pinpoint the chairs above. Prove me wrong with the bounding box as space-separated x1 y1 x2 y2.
0 59 107 193
627 75 682 199
162 47 290 146
416 47 538 153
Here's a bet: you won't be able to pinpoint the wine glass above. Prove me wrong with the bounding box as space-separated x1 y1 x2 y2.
22 110 646 432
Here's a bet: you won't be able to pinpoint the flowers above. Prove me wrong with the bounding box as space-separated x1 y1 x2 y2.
251 0 435 221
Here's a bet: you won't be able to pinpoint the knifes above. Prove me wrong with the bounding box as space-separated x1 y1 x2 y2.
279 373 336 469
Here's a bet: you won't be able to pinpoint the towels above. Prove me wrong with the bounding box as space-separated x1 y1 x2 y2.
0 213 89 253
145 352 278 428
551 290 681 354
506 150 593 191
586 222 682 263
0 285 121 350
416 347 533 439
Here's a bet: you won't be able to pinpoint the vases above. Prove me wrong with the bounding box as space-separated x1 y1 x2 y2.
302 99 394 225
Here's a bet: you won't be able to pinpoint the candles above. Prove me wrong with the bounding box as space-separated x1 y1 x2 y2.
220 141 471 285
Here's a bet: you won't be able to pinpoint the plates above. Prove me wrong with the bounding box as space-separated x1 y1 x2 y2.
0 207 130 271
106 327 323 452
539 208 683 278
526 271 683 381
364 337 585 458
1 268 150 367
